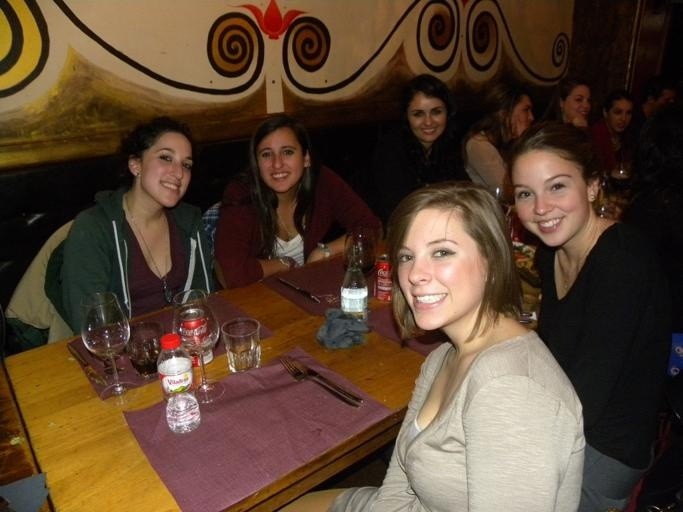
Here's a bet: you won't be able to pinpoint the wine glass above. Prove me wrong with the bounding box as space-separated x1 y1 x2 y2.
172 288 224 405
488 184 512 215
590 163 637 221
344 228 378 279
79 292 142 406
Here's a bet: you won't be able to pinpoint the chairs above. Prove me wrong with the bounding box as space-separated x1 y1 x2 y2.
203 202 227 290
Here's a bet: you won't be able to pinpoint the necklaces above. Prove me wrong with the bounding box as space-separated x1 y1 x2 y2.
123 194 174 305
277 211 299 239
556 223 600 295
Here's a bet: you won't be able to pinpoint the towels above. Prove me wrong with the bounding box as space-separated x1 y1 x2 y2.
318 311 369 348
123 347 391 511
261 250 398 315
66 293 272 399
366 303 448 356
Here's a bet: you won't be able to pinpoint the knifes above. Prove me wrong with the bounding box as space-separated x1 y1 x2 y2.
289 358 363 403
67 344 106 385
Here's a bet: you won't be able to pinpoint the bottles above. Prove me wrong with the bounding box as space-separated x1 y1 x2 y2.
156 334 201 435
341 243 369 333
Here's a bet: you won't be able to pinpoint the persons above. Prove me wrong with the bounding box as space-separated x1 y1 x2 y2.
537 76 593 132
272 179 585 511
507 117 670 512
213 112 385 290
59 116 212 336
353 72 463 240
587 88 640 182
459 79 536 204
620 104 683 333
620 70 678 156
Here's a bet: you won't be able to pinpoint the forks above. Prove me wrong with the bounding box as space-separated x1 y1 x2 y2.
277 277 321 305
281 356 360 409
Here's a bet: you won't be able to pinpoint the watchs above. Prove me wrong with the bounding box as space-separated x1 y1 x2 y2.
317 242 332 261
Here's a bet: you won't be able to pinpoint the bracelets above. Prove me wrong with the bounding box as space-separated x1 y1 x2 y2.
279 256 292 274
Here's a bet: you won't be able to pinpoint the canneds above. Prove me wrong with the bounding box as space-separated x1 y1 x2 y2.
371 253 393 301
177 307 214 367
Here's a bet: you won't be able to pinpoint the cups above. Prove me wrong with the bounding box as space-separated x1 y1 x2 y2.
126 319 165 377
221 318 264 375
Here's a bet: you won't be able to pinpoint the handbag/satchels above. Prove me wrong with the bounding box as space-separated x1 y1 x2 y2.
626 417 683 512
